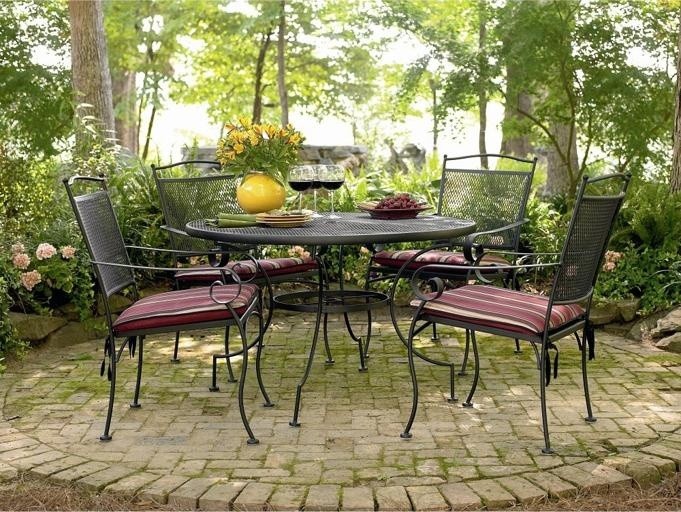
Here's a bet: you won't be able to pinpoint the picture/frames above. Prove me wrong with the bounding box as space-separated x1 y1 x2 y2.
232 170 287 215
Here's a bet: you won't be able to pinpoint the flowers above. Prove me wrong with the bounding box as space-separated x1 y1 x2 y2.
214 113 309 190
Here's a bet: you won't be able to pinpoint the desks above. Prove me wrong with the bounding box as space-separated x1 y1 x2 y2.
183 212 480 428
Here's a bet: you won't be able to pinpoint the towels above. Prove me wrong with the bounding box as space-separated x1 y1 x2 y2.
202 212 262 228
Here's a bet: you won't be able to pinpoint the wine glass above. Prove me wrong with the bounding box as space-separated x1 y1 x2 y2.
289 166 315 213
319 165 346 219
303 164 328 218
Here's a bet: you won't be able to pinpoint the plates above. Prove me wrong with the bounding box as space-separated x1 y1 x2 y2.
356 204 433 218
255 212 314 229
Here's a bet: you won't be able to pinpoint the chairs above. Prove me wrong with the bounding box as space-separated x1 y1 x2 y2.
391 168 634 458
358 150 543 383
62 173 279 448
148 156 335 381
